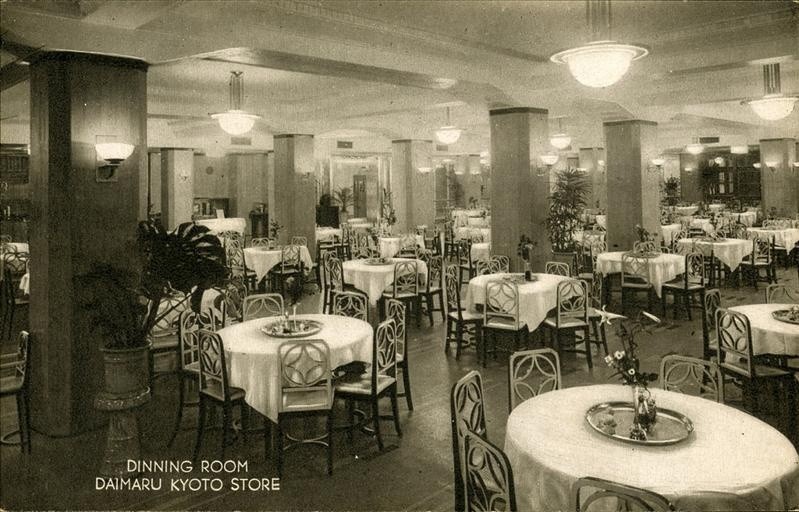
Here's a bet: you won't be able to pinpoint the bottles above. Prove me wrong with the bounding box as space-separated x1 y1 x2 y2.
283 311 290 333
634 396 650 425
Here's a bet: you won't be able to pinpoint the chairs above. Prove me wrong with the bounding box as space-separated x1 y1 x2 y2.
1 199 799 510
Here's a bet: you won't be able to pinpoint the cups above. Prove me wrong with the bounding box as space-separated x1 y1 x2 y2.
372 245 379 262
524 262 531 281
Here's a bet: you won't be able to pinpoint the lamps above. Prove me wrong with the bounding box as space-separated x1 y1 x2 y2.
547 117 574 151
432 104 465 147
89 132 137 185
208 68 264 137
548 1 652 92
652 131 799 174
533 150 560 178
736 62 799 123
